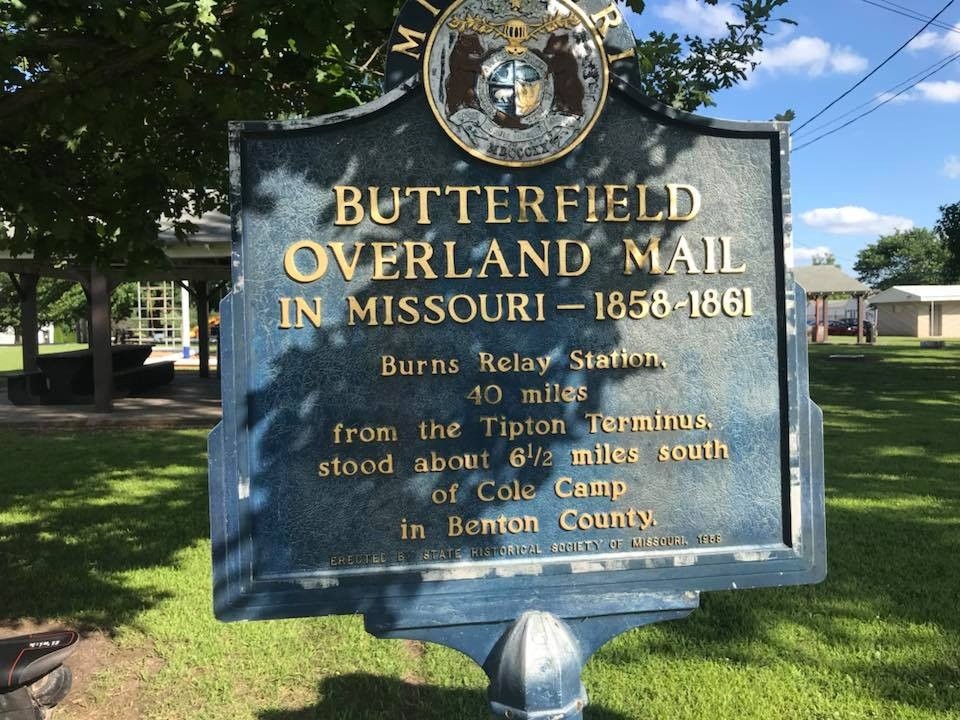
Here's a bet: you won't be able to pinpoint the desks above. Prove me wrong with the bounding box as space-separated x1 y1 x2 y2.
34 344 156 406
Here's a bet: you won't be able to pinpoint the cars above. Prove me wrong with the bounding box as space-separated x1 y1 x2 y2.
809 320 867 337
839 318 874 330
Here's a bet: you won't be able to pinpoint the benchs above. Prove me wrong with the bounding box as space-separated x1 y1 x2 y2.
0 370 44 404
114 361 174 393
